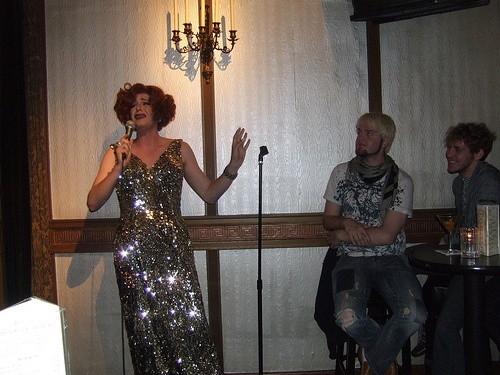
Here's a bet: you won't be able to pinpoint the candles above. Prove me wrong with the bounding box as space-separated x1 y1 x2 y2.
198 0 205 26
230 0 236 30
173 0 179 30
214 0 220 21
184 0 190 23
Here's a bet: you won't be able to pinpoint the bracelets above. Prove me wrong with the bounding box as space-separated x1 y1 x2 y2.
108 171 117 185
223 167 238 181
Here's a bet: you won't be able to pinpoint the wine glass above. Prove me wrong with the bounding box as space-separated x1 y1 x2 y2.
436 214 462 253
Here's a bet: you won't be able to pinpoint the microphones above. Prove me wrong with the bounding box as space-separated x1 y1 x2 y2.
122 120 136 160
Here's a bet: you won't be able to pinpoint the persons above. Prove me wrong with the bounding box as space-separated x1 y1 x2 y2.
409 121 500 375
322 111 432 375
84 81 252 375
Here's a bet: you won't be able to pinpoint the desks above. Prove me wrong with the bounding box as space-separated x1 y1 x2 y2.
404 244 500 375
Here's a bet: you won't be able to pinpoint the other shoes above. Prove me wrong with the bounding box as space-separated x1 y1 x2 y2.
357 347 372 375
412 340 427 358
386 359 398 375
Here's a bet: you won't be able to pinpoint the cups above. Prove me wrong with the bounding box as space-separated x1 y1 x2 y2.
459 227 481 258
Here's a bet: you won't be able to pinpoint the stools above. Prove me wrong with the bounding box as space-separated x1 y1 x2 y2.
335 304 412 375
